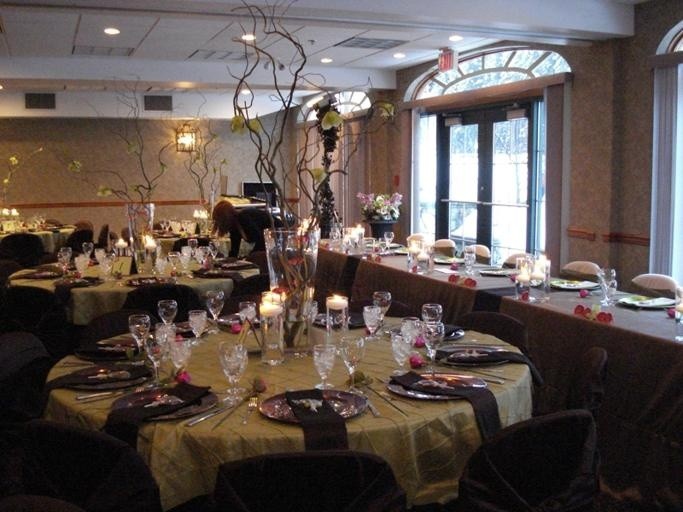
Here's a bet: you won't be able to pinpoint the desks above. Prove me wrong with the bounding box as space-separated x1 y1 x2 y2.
45 314 533 508
7 256 261 333
316 238 682 447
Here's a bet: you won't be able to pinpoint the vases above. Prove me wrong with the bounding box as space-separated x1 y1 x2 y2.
124 200 155 239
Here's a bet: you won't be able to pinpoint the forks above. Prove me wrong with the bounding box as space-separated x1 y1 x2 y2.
241 396 257 425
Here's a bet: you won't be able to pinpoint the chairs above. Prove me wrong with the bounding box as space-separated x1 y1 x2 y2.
500 251 535 268
122 282 201 324
461 244 491 264
457 411 601 511
6 287 79 355
456 312 529 354
1 219 224 277
6 424 161 512
627 272 682 301
214 451 408 510
221 293 263 315
348 302 413 318
82 311 160 349
230 273 271 293
247 251 267 272
430 240 457 258
559 262 606 286
550 376 604 453
6 319 52 421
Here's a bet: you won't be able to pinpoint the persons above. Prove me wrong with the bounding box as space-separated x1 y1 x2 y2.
211 200 283 269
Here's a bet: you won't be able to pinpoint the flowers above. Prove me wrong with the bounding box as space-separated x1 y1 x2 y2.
353 186 403 222
221 0 394 348
0 142 47 206
180 90 227 218
57 68 175 231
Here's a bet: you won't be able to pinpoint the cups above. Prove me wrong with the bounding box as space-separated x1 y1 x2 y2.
0 215 44 234
328 223 552 303
158 218 196 238
674 287 683 341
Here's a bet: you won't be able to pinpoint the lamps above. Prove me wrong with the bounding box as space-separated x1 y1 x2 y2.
175 122 196 153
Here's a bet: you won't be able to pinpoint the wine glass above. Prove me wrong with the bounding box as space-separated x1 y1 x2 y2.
372 289 391 312
57 238 219 276
385 303 443 375
308 313 335 388
215 341 249 398
360 305 382 346
239 301 256 338
203 290 225 333
182 309 208 347
167 337 190 376
157 300 178 324
153 323 176 369
598 266 617 305
128 314 150 352
338 335 364 389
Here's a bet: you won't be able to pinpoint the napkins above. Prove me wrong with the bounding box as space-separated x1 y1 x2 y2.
67 342 144 358
286 388 350 454
35 364 151 421
55 275 100 313
390 371 503 441
447 351 544 415
105 382 210 453
441 324 464 337
203 272 243 285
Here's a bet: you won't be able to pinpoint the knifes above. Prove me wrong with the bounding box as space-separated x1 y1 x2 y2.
350 386 378 416
185 404 233 427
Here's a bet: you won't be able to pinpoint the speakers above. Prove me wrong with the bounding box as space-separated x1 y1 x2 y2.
242 181 278 207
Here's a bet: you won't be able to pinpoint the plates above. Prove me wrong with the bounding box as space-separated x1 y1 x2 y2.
260 389 368 424
388 373 487 400
110 388 218 421
65 366 147 390
73 343 140 358
549 280 600 291
436 345 511 367
125 277 176 287
218 260 256 270
196 270 239 278
619 296 676 310
52 277 103 288
390 326 464 343
